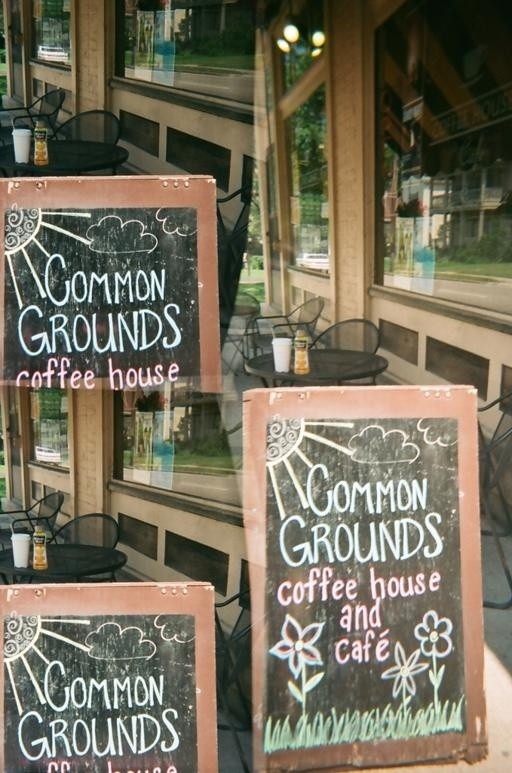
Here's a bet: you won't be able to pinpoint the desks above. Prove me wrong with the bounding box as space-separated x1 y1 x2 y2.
244 349 388 387
1 140 129 175
0 544 128 585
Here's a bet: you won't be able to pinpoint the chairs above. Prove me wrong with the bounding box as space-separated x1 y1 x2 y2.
222 293 260 376
0 88 66 139
308 319 382 354
476 392 512 610
215 589 251 773
43 513 120 549
0 492 64 551
48 110 121 144
252 296 324 357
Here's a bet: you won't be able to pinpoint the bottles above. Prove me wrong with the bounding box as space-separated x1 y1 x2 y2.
292 329 310 374
33 526 48 571
32 120 50 166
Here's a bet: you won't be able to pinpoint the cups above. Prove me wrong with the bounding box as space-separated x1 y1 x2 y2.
270 337 293 373
10 127 33 163
11 533 31 568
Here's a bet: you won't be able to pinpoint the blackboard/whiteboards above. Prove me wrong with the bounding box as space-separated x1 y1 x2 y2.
0 174 224 394
242 384 487 773
1 581 219 773
